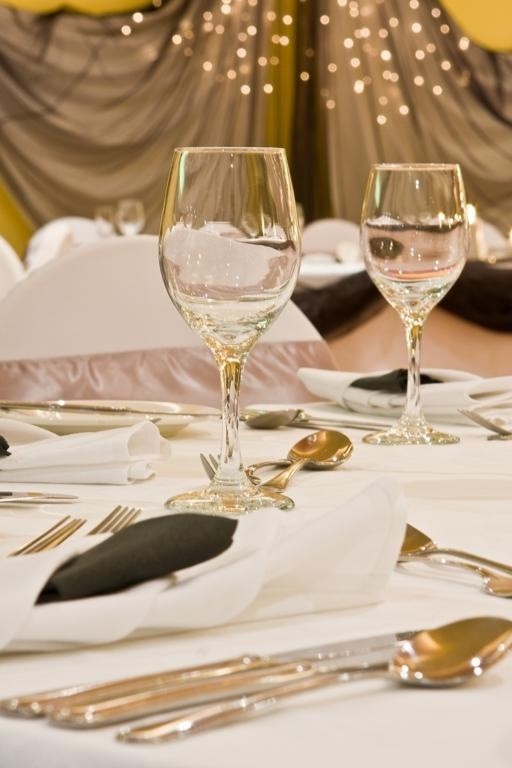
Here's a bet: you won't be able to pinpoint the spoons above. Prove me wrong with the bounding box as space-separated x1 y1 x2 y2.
115 612 512 750
236 407 302 428
397 554 512 601
256 428 353 496
398 521 512 574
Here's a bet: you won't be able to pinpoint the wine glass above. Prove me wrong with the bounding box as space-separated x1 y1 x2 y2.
358 160 470 448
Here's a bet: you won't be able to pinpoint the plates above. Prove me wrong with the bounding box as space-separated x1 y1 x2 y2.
152 145 303 517
0 399 222 436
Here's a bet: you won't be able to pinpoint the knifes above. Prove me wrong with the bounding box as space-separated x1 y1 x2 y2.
53 645 393 735
1 401 221 416
0 490 78 501
2 629 422 717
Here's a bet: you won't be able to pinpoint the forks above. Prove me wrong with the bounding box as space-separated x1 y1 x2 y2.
0 514 89 554
455 408 512 435
200 446 292 484
81 503 142 537
